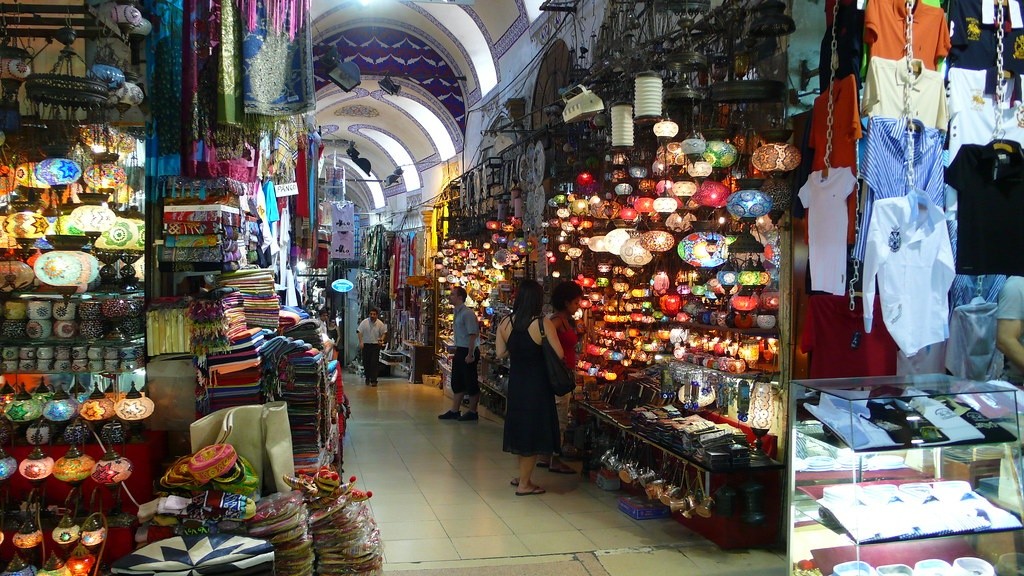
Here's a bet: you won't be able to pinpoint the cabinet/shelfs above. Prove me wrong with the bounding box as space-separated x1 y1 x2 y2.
790 371 1024 576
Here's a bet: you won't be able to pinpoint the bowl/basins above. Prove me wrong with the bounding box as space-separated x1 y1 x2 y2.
0 300 145 338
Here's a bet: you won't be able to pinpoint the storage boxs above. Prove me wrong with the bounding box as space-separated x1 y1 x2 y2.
617 496 671 520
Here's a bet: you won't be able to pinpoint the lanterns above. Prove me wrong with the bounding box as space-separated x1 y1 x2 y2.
0 374 155 576
1 3 155 292
543 117 802 378
487 218 500 231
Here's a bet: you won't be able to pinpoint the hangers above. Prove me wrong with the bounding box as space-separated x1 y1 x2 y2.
850 278 863 298
975 286 982 295
998 67 1012 80
911 187 928 212
994 0 1007 5
903 42 920 74
904 111 920 132
820 157 829 178
994 129 1014 154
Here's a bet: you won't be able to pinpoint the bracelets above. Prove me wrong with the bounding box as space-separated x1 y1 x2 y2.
467 353 475 360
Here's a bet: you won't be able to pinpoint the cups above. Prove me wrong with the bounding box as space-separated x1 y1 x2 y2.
0 343 145 371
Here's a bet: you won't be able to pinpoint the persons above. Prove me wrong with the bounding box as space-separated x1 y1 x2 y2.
997 275 1024 391
301 294 328 328
536 280 584 475
357 308 387 387
495 279 565 496
328 317 341 360
437 287 482 423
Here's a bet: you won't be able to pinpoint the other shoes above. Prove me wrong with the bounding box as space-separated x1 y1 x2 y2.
365 380 370 385
372 382 377 387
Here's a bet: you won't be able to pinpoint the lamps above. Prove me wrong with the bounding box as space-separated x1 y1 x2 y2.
0 0 152 307
318 44 361 93
385 165 404 187
0 366 153 576
347 140 371 177
377 72 402 96
436 0 802 401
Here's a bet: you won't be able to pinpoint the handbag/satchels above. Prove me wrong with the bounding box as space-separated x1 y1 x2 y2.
538 317 576 397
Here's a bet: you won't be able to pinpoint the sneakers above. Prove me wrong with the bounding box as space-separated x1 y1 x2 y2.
457 410 479 420
438 410 461 420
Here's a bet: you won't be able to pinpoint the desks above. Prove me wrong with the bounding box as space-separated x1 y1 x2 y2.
575 399 785 551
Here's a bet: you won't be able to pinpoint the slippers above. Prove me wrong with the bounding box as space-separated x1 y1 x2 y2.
511 481 520 485
515 485 546 495
536 463 550 467
549 466 576 474
